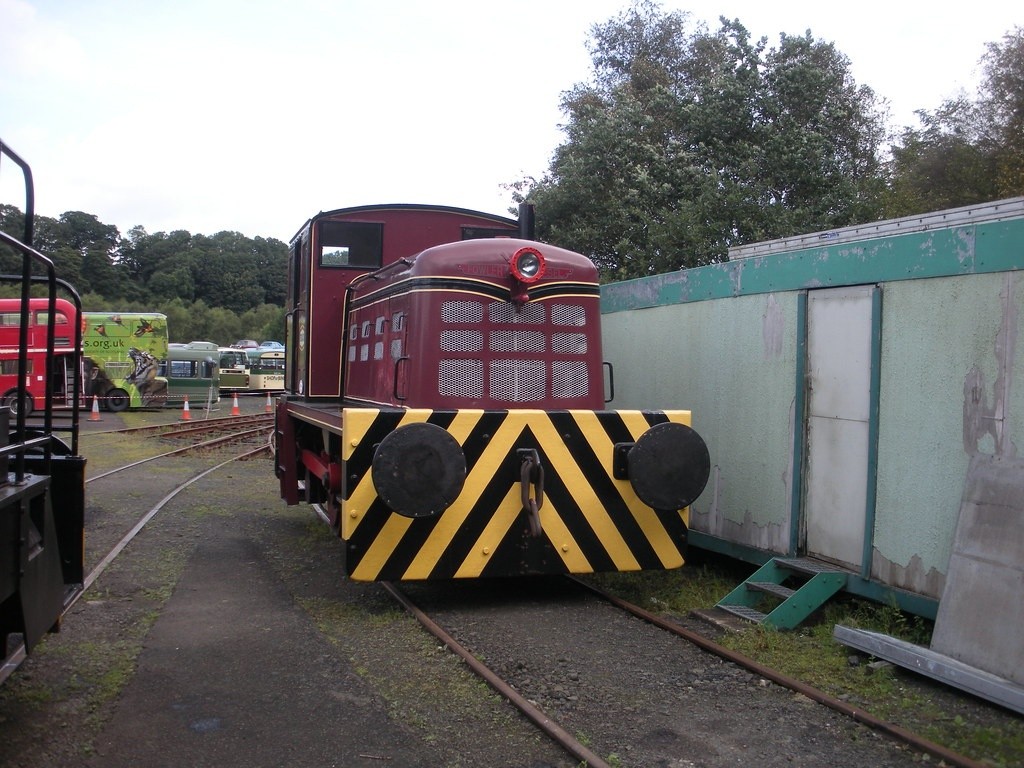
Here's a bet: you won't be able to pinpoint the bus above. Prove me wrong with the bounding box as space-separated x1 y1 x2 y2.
168 343 286 408
1 299 87 419
1 311 169 412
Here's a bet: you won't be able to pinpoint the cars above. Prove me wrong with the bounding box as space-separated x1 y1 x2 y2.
257 341 282 351
230 340 259 350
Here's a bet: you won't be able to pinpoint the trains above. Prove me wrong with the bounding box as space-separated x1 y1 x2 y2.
276 202 711 581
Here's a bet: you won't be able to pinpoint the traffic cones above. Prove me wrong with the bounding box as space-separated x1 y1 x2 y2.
86 394 104 422
229 392 242 416
265 391 273 412
177 394 192 421
